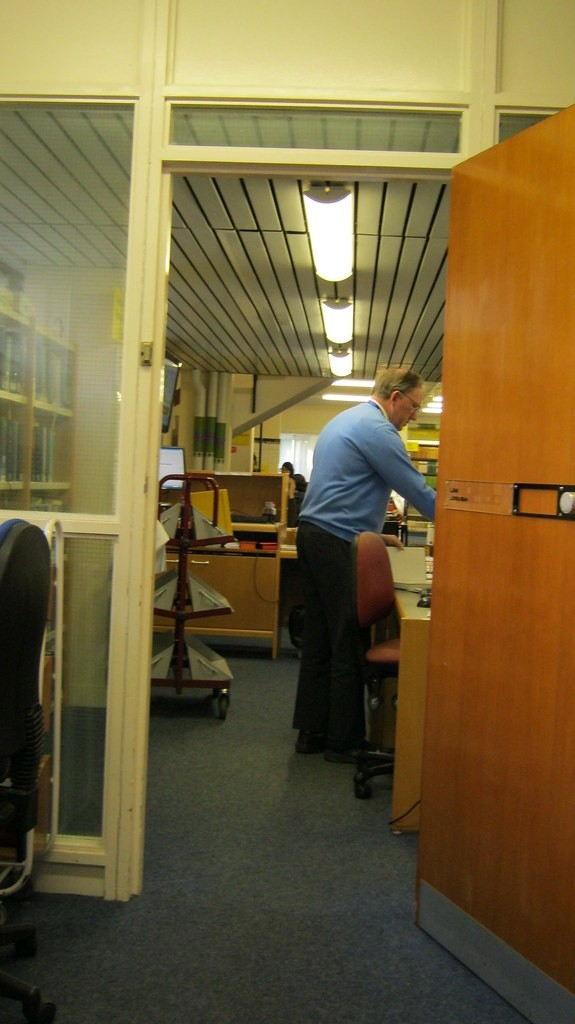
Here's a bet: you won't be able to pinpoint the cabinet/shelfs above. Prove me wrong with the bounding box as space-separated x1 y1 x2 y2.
151 473 238 719
152 469 289 658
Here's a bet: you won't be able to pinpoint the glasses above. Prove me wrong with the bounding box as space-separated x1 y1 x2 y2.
403 393 421 411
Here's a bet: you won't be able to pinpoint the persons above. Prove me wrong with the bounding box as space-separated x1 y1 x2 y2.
279 463 296 500
293 365 445 763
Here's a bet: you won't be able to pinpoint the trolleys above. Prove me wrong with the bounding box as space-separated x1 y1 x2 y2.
149 473 235 722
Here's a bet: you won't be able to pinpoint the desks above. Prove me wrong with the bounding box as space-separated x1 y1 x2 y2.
370 588 431 835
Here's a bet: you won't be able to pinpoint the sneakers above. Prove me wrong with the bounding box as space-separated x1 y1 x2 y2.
326 739 375 762
296 731 329 753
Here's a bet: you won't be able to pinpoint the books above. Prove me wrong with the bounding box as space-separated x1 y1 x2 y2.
2 315 72 514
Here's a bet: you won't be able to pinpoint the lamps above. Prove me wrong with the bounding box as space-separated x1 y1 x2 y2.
328 348 353 377
321 298 354 344
301 185 354 283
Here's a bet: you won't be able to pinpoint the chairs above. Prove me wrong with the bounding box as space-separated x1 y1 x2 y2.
0 517 57 1024
348 529 401 800
